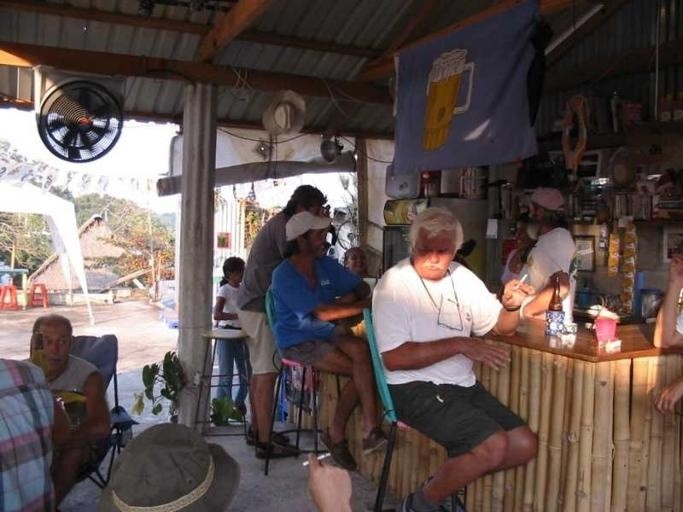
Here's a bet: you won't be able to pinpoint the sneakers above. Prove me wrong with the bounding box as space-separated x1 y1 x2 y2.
247 425 301 458
397 476 467 512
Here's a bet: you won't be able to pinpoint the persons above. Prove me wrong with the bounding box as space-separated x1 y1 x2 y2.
1 359 65 512
263 208 391 473
500 210 537 286
649 250 681 420
92 422 355 512
367 202 541 512
341 243 369 279
500 185 579 324
21 312 111 510
213 256 248 420
236 181 328 461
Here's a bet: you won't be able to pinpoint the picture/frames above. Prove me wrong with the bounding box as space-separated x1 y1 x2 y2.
662 227 683 263
574 234 595 273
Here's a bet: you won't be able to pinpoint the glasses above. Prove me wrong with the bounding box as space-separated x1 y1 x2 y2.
437 295 463 331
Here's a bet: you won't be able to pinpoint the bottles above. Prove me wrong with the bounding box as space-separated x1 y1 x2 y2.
545 273 564 333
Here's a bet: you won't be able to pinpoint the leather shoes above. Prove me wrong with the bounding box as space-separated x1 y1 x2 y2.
362 428 390 455
320 426 357 471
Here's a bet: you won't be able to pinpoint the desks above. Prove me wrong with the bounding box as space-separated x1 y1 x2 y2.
574 304 632 322
0 268 29 310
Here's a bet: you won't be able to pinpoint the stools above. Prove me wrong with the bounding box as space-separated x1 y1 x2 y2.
28 284 48 309
194 328 249 442
0 284 18 310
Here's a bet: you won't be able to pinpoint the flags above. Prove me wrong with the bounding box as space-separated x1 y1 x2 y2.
391 4 540 176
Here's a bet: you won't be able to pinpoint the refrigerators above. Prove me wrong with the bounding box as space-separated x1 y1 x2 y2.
379 199 487 286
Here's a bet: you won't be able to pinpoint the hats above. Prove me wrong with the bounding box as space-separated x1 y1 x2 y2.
98 422 239 512
531 187 565 211
285 211 333 241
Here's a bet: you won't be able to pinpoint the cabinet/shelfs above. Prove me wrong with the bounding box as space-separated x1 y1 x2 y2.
537 0 682 149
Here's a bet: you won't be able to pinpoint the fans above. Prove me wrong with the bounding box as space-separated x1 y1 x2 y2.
36 77 123 163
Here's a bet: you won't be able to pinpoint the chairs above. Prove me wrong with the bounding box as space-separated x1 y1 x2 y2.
264 285 341 476
30 334 139 489
362 307 468 512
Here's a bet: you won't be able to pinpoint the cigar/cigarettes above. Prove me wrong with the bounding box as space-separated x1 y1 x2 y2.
298 453 333 467
515 272 529 290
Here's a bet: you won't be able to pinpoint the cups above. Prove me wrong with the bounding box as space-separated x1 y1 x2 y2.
594 317 617 344
